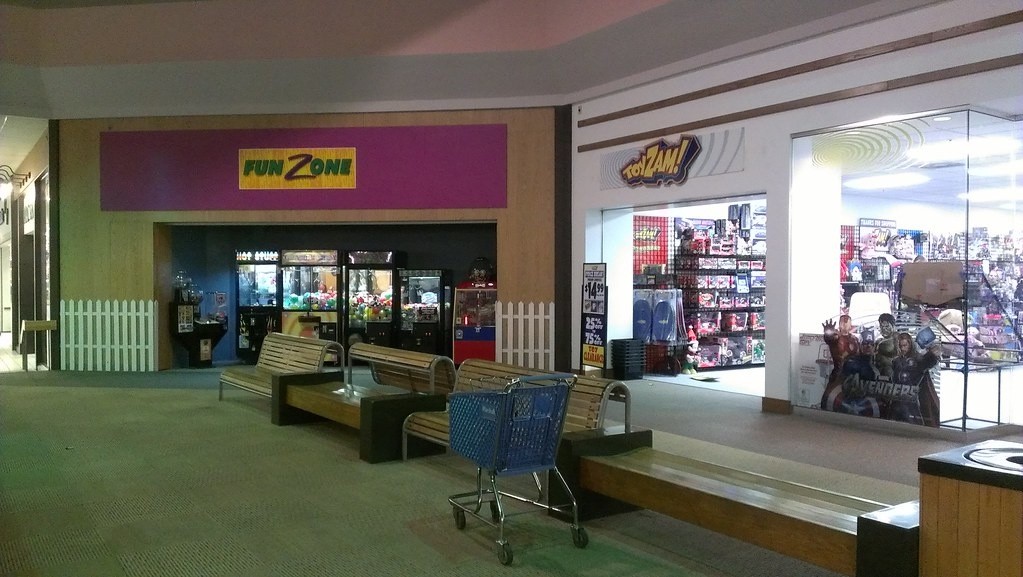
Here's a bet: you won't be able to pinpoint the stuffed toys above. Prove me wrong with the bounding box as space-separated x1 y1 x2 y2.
890 235 906 259
938 309 973 342
860 234 878 260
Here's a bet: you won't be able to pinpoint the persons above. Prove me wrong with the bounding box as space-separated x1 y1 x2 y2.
821 315 860 412
843 313 940 427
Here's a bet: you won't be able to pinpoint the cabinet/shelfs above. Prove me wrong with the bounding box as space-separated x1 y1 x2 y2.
674 253 766 372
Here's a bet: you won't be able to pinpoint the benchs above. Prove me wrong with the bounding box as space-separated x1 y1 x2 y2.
271 370 446 462
403 358 633 462
549 425 919 577
348 342 456 396
219 332 344 401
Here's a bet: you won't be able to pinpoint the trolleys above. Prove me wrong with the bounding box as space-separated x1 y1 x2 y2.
447 370 590 565
